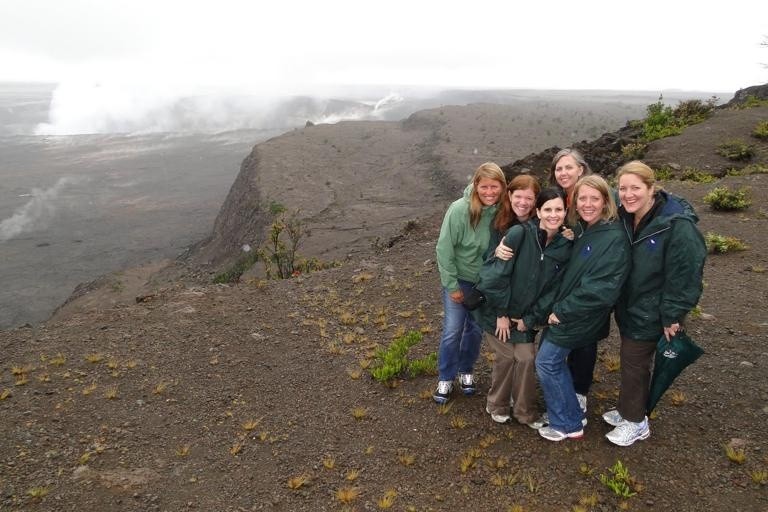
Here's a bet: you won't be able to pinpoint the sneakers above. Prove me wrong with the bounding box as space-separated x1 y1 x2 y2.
432 380 454 403
485 407 513 424
542 412 587 427
523 417 544 428
577 393 587 411
602 409 621 426
458 374 476 392
538 425 584 441
605 416 650 446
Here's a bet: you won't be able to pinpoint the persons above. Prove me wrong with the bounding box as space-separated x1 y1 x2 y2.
433 162 516 405
535 174 632 442
476 186 576 429
602 161 707 447
537 149 598 414
482 175 540 267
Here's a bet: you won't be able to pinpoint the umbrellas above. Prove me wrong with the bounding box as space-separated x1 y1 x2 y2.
645 324 705 417
